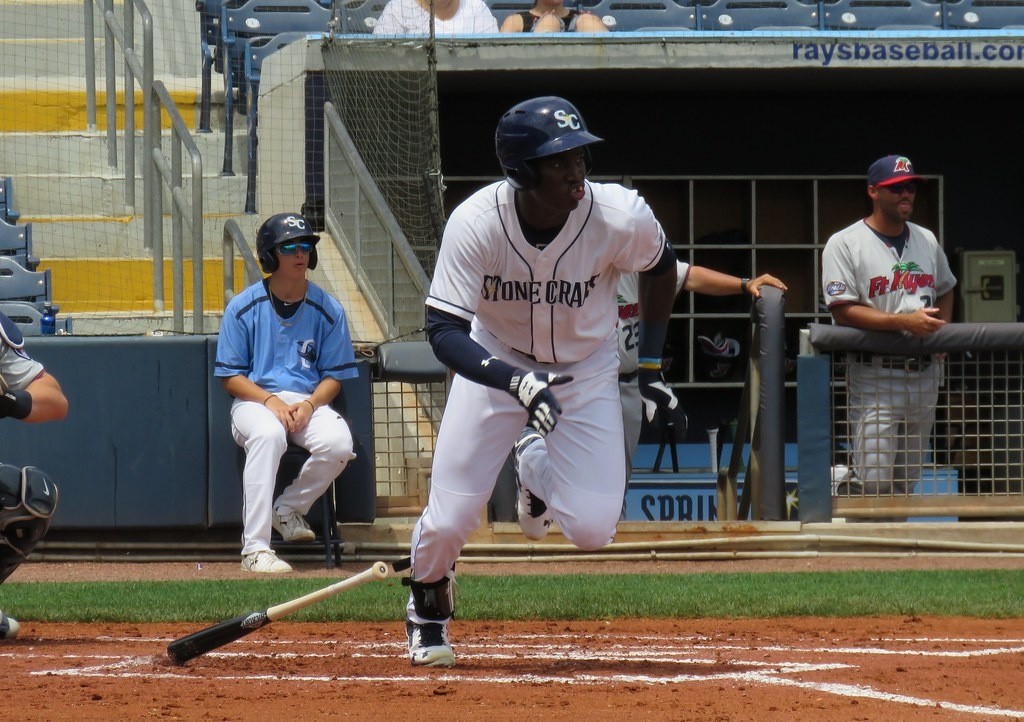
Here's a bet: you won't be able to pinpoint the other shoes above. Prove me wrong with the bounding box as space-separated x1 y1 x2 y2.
0 611 20 642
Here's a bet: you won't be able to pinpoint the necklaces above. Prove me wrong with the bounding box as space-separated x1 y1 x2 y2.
269 280 307 326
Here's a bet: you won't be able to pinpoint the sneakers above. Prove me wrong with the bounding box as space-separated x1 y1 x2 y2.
511 427 554 541
403 617 455 668
273 500 315 542
240 550 293 573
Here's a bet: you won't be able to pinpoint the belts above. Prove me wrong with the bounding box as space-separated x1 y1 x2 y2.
487 328 555 366
854 353 932 373
618 372 636 383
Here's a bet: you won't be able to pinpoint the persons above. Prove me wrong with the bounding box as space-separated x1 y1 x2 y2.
616 259 788 523
0 310 68 642
501 0 609 32
213 214 358 574
372 0 498 34
405 95 688 668
821 154 958 523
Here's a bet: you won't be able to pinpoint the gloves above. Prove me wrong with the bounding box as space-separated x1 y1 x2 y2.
509 368 574 438
637 368 688 432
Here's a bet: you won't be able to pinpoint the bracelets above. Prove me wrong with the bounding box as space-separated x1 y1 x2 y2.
305 400 314 412
741 278 749 294
264 395 276 405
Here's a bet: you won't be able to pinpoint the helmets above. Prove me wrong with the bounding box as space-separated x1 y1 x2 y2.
495 96 605 193
698 336 744 381
256 213 320 275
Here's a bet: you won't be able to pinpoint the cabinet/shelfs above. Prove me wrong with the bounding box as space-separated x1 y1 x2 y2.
378 172 944 386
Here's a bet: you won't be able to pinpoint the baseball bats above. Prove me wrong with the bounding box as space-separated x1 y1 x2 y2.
167 561 389 662
706 425 720 473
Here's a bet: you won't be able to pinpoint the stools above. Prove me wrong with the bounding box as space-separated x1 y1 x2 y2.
272 445 343 570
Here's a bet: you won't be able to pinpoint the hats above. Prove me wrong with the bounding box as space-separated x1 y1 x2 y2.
867 154 925 186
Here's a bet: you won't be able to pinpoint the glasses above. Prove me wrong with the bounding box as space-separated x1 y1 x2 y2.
883 181 918 194
275 242 313 255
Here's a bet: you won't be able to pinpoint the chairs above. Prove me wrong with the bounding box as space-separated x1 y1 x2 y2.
0 177 73 337
195 0 1024 216
